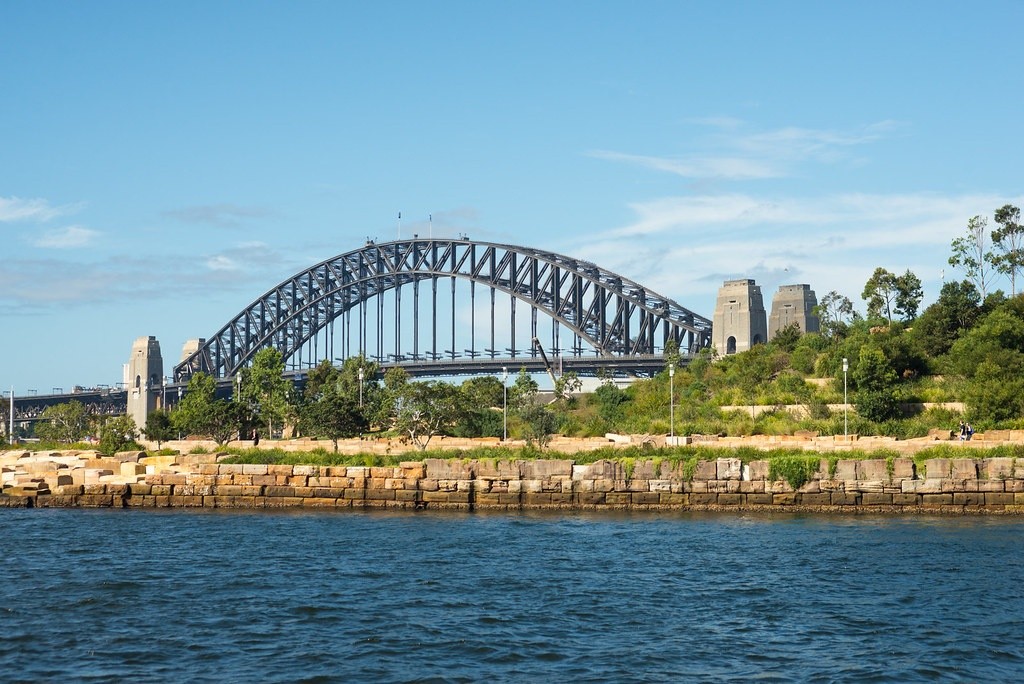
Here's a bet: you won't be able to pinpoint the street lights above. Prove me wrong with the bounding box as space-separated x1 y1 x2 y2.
177 387 183 442
162 379 167 416
843 358 850 436
501 367 509 439
236 372 241 442
668 363 674 437
359 368 365 407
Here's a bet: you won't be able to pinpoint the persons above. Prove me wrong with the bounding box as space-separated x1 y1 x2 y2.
959 421 973 440
252 428 259 448
